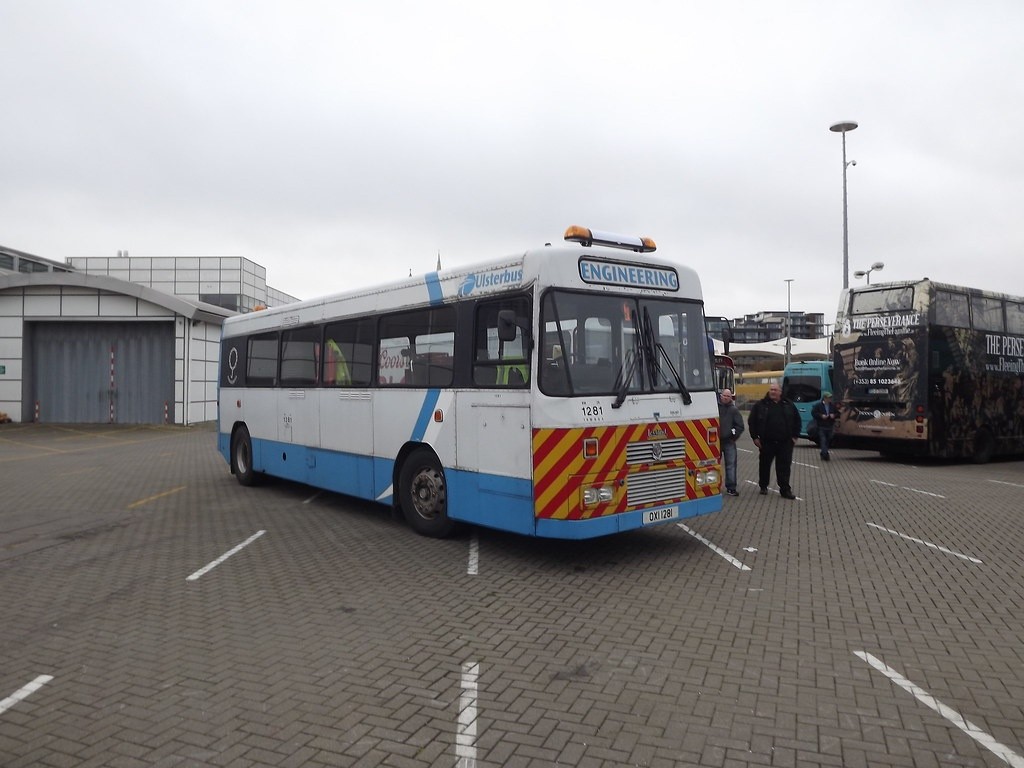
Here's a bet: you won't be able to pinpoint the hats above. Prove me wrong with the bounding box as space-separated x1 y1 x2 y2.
823 392 832 398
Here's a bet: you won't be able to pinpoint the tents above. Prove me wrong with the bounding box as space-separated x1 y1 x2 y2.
711 336 833 386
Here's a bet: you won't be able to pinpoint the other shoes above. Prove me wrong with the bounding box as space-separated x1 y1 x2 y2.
820 453 830 461
781 490 796 499
760 486 767 495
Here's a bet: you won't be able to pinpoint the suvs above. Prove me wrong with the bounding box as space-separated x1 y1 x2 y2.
832 277 1024 465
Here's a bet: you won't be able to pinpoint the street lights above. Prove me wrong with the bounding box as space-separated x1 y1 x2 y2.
854 262 885 284
829 119 857 289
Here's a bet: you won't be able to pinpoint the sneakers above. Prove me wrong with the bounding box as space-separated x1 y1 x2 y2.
726 489 739 496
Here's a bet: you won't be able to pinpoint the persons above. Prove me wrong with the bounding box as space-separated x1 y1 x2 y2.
810 391 840 461
748 383 801 499
718 388 745 496
834 333 919 420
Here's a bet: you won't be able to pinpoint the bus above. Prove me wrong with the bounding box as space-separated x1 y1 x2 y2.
217 226 724 541
713 355 736 406
783 360 836 449
734 370 784 401
